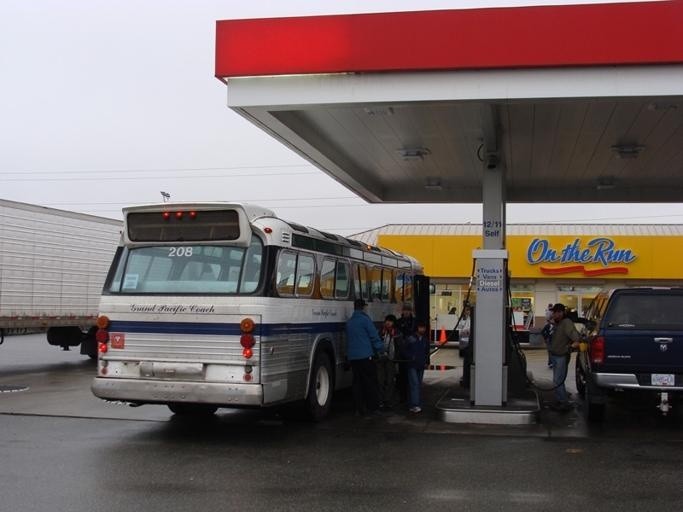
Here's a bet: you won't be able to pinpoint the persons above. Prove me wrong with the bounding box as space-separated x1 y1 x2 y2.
344 298 385 421
401 321 432 416
545 303 553 318
542 317 558 368
548 303 581 409
377 315 404 410
397 302 418 334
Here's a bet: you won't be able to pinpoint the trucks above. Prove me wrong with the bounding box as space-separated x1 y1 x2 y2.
0 198 125 365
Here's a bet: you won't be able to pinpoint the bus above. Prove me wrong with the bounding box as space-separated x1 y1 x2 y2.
91 200 437 428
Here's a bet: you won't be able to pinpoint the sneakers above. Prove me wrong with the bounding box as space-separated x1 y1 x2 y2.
408 406 423 413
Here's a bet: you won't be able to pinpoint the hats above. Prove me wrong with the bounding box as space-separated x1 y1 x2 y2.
354 298 368 307
549 303 566 312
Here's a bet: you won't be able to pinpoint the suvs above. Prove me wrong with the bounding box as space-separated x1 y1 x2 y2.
569 286 683 431
